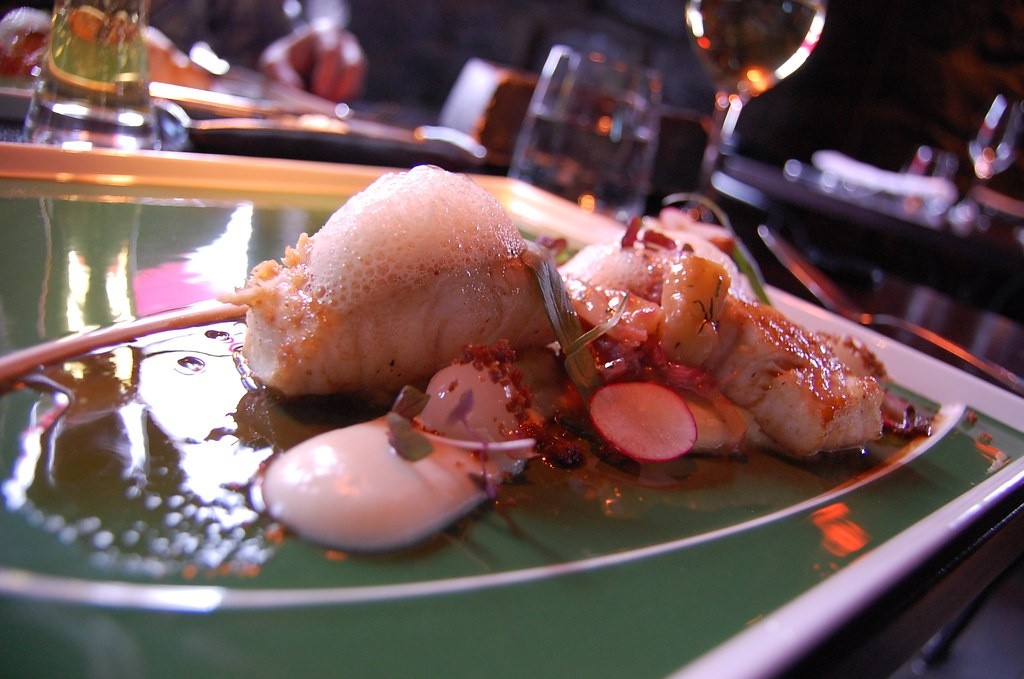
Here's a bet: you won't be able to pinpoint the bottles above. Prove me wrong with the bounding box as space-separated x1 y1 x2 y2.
22 0 161 151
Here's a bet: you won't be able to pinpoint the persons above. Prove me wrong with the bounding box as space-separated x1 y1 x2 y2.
143 0 366 101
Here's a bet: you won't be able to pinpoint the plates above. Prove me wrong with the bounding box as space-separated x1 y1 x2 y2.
0 143 1024 679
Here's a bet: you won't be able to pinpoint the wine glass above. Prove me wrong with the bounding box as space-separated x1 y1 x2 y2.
949 92 1024 232
683 0 826 227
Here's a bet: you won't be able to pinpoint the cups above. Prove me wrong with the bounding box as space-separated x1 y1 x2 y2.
506 42 667 228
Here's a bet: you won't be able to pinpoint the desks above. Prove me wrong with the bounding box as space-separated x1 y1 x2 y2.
711 157 1023 330
0 143 1024 679
